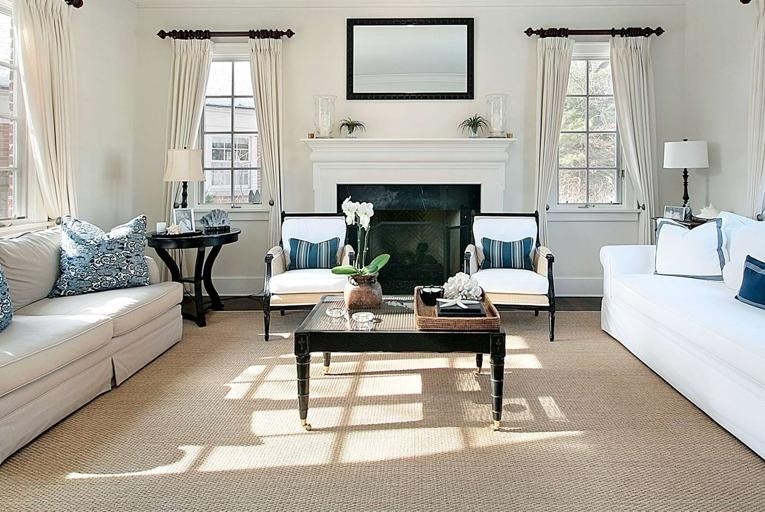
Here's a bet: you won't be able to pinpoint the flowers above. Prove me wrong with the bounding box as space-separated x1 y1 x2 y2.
332 195 390 276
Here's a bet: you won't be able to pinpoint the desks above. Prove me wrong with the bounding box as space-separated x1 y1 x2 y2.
146 229 240 327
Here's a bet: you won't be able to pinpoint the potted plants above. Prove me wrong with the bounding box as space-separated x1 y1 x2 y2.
455 114 490 139
337 115 367 139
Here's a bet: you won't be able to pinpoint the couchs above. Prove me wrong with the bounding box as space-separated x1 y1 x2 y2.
600 211 763 464
0 224 184 466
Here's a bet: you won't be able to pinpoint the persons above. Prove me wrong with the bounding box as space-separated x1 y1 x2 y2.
178 213 191 230
675 209 684 219
666 209 674 217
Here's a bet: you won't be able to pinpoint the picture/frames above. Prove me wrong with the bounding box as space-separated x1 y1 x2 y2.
344 15 477 102
174 208 195 232
664 206 685 221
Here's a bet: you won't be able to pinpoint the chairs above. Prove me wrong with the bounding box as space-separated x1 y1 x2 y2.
460 209 557 341
264 210 357 344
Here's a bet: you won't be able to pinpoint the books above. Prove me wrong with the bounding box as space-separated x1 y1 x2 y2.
439 300 482 312
434 304 487 318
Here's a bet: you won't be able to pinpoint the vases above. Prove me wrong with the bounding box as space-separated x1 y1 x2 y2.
344 276 382 309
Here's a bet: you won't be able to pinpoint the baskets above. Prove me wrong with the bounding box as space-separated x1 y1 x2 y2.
413 283 501 332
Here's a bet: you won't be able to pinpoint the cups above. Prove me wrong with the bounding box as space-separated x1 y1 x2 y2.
419 286 444 307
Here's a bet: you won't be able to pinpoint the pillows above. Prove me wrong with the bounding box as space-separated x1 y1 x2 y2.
49 211 150 298
286 236 339 271
0 261 16 329
649 211 731 282
722 251 763 307
479 236 534 270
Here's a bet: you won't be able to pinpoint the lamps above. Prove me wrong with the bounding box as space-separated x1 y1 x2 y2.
164 147 207 206
663 137 710 211
